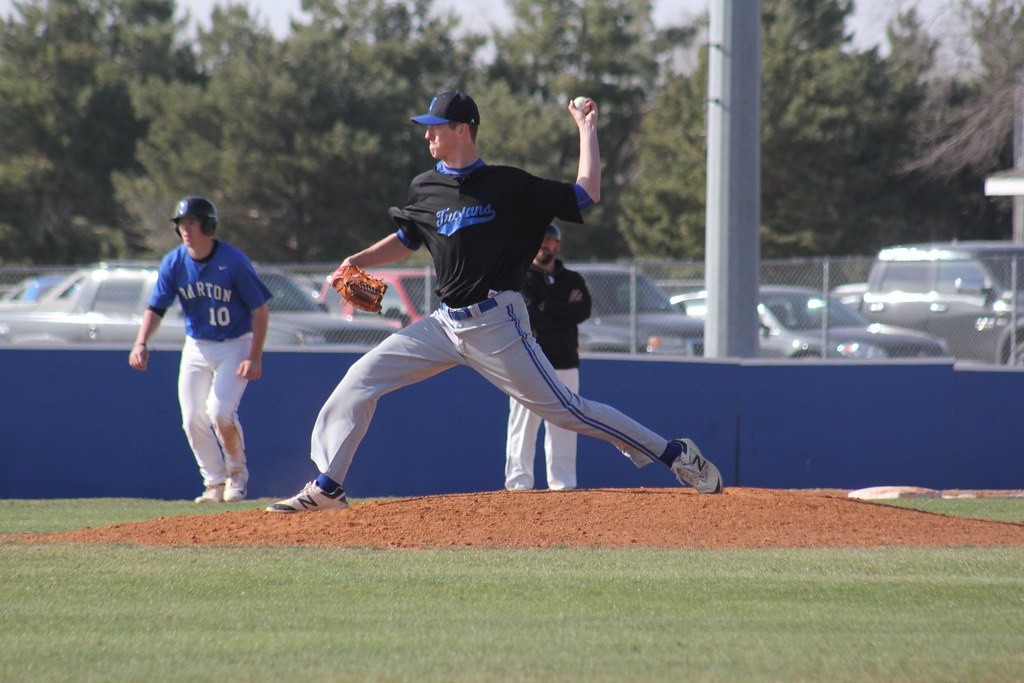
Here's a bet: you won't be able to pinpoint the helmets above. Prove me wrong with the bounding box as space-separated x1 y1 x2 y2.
170 196 219 237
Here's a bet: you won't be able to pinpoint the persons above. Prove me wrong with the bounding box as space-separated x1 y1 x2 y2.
128 195 274 503
265 89 724 512
504 223 591 491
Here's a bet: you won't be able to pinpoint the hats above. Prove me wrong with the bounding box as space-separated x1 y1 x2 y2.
410 91 481 128
544 224 562 241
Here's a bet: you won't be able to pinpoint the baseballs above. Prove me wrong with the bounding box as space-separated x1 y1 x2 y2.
573 95 591 114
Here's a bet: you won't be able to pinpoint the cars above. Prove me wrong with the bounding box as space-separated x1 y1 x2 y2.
320 270 440 331
562 265 705 357
0 270 402 355
668 288 950 362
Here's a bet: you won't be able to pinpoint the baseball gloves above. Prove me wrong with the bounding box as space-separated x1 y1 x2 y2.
329 264 389 313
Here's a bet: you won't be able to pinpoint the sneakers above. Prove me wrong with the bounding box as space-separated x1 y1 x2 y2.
265 480 347 513
668 438 723 496
195 475 248 502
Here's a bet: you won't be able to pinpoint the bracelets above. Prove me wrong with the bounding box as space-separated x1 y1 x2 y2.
137 343 147 345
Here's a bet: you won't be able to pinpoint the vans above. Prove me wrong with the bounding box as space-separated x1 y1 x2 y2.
860 242 1024 366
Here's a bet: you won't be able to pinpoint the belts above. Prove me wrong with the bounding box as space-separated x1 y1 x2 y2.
440 300 498 321
